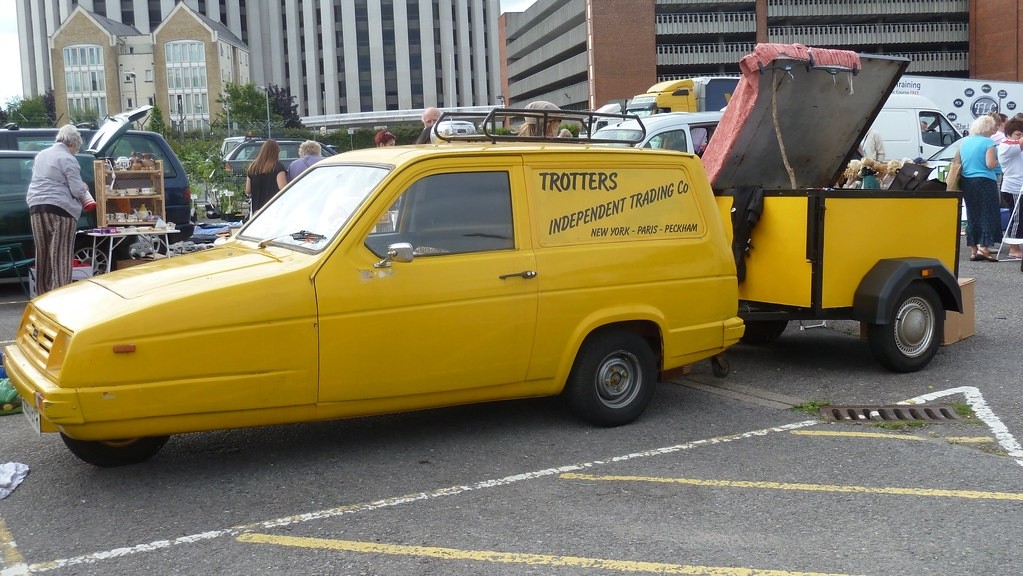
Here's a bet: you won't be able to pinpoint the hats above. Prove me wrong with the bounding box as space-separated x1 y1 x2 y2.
525 100 564 124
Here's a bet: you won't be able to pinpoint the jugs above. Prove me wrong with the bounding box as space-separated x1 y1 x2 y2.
133 204 152 221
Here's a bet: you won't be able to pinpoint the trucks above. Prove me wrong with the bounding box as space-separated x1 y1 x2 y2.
578 99 633 138
623 76 744 149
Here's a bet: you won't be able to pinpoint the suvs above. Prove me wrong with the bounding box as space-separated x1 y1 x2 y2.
204 131 339 224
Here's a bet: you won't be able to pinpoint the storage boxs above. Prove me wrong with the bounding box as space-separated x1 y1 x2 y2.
939 278 976 345
29 266 93 299
117 258 153 270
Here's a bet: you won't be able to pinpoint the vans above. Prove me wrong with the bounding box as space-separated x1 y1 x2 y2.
590 111 727 159
0 121 197 256
858 93 969 220
219 136 288 160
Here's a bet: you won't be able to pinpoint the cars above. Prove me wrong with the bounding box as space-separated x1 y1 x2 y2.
2 108 746 466
0 105 154 283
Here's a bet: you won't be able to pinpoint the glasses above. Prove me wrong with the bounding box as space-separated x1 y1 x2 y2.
379 131 391 145
994 123 1001 127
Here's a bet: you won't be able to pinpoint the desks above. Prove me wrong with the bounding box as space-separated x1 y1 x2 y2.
87 228 181 275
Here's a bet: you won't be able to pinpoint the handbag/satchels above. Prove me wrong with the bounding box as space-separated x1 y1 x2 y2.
946 145 962 192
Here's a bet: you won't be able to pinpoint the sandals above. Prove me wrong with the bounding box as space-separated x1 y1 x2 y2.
970 249 998 262
1006 249 1023 259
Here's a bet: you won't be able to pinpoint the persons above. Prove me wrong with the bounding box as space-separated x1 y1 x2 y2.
287 139 325 182
27 125 90 296
955 111 1023 262
375 130 396 148
511 101 563 138
859 131 886 164
245 138 287 216
415 107 441 145
559 129 573 138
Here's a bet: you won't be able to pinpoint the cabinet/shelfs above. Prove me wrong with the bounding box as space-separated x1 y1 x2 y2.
94 159 166 229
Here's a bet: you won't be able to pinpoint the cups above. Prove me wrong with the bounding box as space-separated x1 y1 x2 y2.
116 189 126 195
126 188 139 194
115 213 127 219
141 187 153 193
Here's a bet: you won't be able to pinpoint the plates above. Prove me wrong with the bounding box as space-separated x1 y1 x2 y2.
125 194 140 196
139 192 157 195
